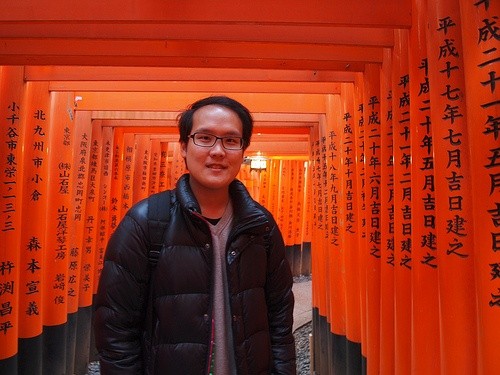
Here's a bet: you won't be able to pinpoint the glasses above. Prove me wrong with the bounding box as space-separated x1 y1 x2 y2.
187 131 245 150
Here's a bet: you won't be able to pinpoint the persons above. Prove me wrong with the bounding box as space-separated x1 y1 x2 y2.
91 96 297 375
